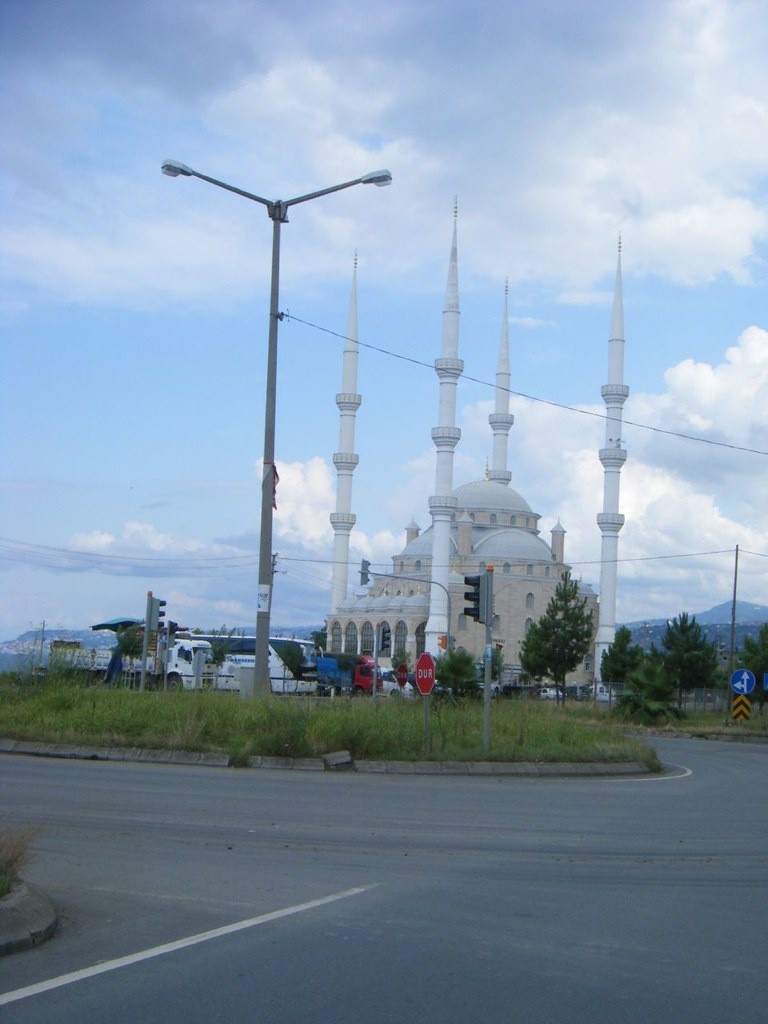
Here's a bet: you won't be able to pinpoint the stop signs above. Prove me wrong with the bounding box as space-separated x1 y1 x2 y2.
417 651 436 695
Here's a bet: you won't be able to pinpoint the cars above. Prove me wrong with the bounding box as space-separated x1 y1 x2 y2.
539 688 563 699
380 668 415 697
401 672 442 695
471 678 501 698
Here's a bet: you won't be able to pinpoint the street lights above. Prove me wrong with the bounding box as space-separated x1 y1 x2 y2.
160 158 395 691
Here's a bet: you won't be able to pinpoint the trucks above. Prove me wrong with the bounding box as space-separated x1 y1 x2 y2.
316 653 384 695
60 637 218 692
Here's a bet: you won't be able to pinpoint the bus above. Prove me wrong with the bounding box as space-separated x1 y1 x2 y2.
182 633 324 698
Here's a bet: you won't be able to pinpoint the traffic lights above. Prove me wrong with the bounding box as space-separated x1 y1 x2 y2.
168 620 179 635
437 636 446 649
150 598 166 630
463 572 488 627
378 626 392 653
